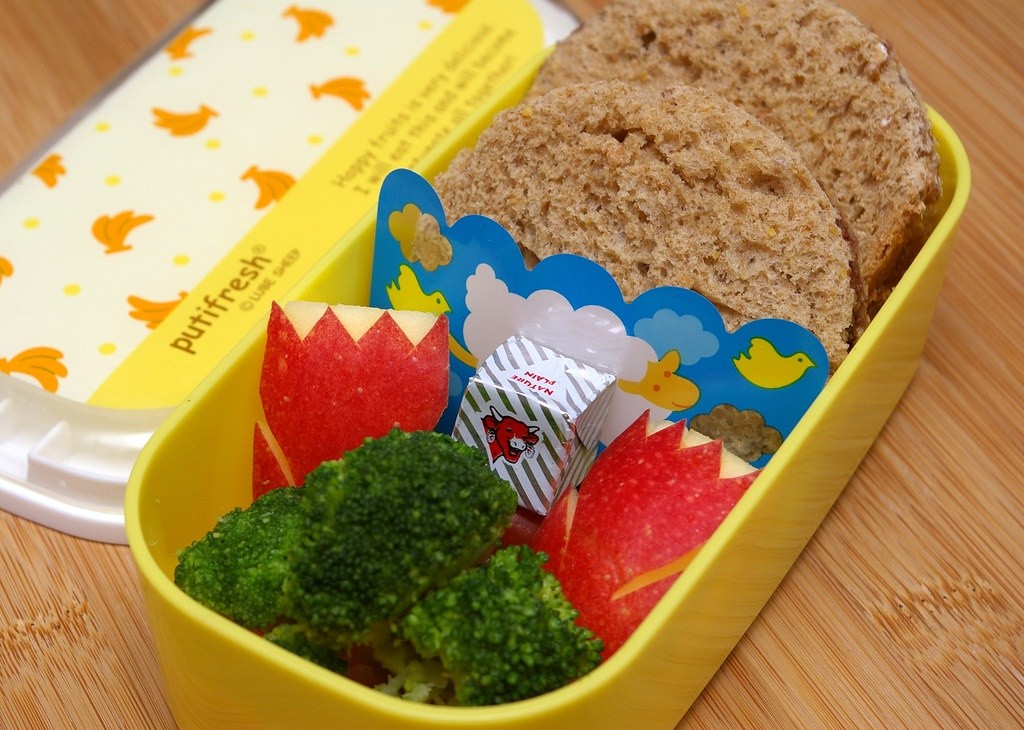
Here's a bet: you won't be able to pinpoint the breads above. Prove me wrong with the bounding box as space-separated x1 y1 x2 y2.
528 0 943 303
435 78 872 374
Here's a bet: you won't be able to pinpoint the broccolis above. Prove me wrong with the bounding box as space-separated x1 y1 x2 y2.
171 426 605 707
